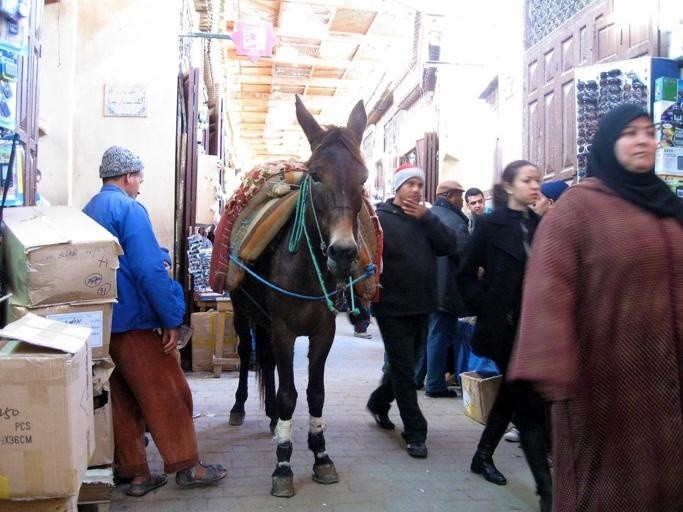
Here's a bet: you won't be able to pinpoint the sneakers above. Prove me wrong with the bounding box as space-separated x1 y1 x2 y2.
353 329 373 340
504 428 520 441
423 388 458 400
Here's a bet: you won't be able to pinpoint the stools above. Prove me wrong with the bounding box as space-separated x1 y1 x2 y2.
213 297 233 379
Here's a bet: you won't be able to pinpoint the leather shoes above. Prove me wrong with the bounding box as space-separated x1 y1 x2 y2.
366 402 395 430
536 488 555 512
469 450 507 486
407 437 428 458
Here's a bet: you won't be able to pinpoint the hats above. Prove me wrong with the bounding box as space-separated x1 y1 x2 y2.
541 178 569 201
99 145 145 178
393 163 425 192
435 180 466 194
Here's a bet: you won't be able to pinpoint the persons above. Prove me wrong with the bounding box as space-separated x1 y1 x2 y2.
533 180 569 218
79 145 229 497
507 102 683 511
365 161 458 459
415 180 472 398
345 305 374 341
465 188 492 232
453 159 552 512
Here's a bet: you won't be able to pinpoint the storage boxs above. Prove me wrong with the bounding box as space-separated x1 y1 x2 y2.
88 363 115 467
2 204 123 309
1 326 97 501
4 287 112 360
190 310 239 373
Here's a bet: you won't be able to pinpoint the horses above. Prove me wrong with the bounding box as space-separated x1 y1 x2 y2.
230 94 368 498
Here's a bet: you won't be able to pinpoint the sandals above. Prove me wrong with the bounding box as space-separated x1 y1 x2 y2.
128 474 167 495
176 463 227 487
